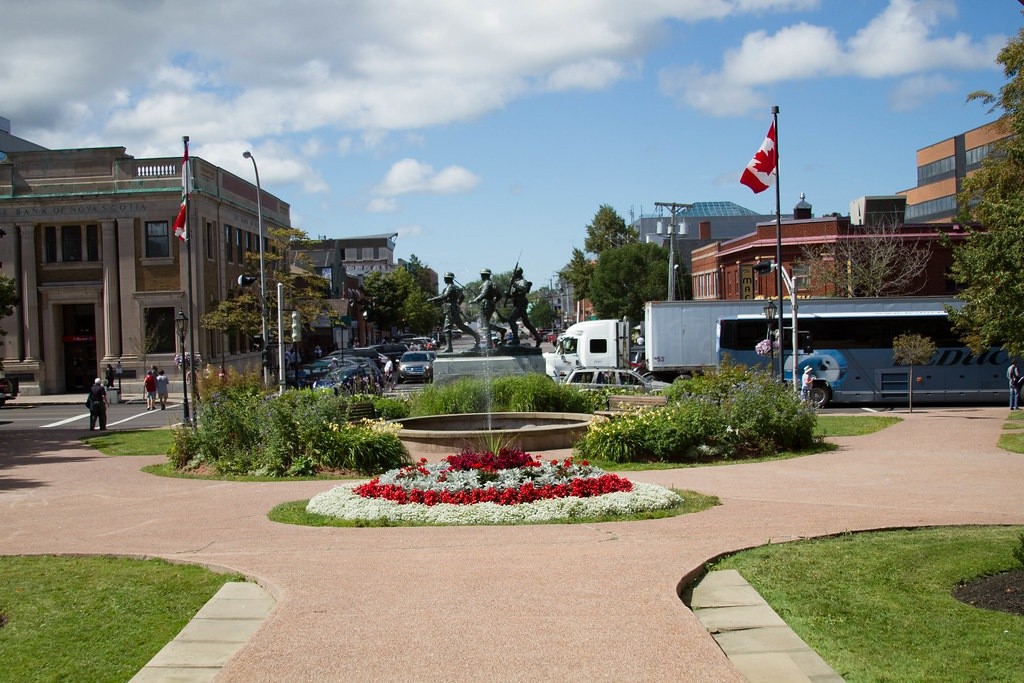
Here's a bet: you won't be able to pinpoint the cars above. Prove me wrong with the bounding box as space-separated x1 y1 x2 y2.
563 367 674 394
0 375 16 405
629 346 645 364
286 334 445 397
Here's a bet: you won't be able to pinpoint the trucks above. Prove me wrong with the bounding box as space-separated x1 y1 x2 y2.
554 295 971 381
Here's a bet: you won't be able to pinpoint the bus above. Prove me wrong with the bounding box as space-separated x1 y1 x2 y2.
716 313 1024 405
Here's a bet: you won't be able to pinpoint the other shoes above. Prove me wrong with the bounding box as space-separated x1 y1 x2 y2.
147 406 150 410
152 406 155 409
1010 407 1014 410
1015 407 1022 410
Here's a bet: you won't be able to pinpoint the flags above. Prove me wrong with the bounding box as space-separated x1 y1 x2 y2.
739 122 776 194
173 145 193 241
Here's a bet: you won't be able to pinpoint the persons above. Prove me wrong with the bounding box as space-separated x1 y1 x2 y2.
504 267 543 347
410 338 437 351
801 365 814 401
88 378 108 430
472 268 507 348
426 272 480 352
285 349 301 364
1006 358 1021 410
106 364 114 387
314 346 322 357
186 371 200 401
384 357 393 391
328 358 337 372
144 365 169 410
205 364 226 379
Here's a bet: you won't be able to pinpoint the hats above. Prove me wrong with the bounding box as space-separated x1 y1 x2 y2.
804 365 813 373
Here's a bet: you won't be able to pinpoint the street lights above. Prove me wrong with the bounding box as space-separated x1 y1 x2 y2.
173 308 189 422
763 300 777 384
242 151 272 390
654 200 695 299
